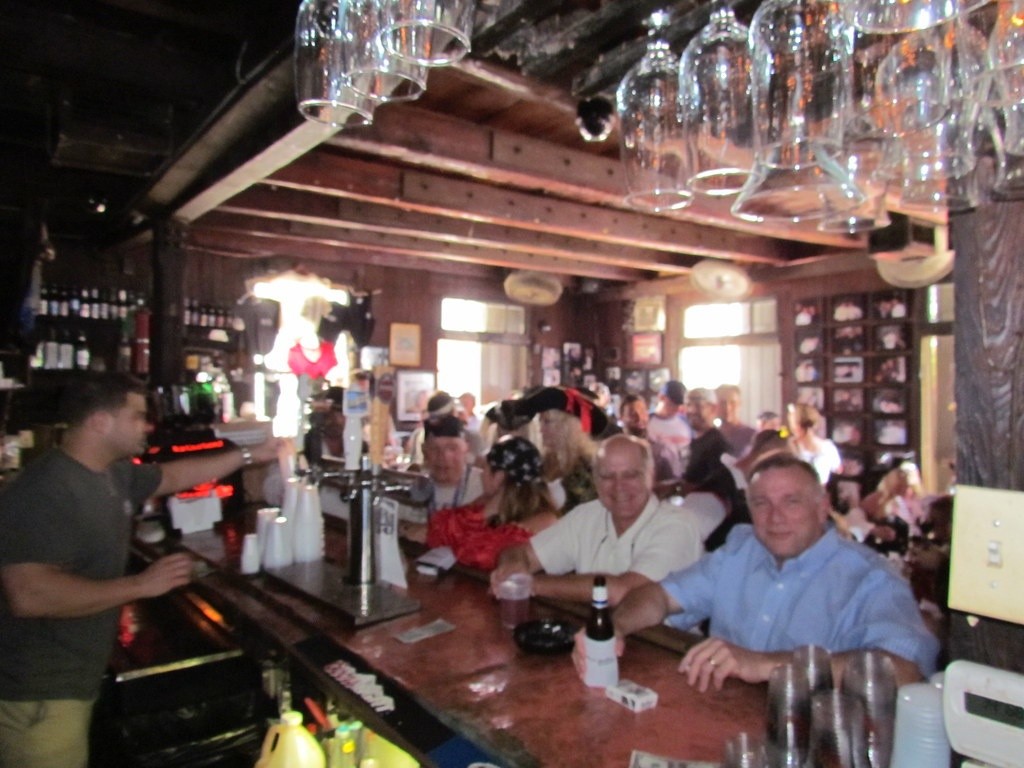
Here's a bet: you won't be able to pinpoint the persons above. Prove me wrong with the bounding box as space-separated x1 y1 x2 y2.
408 414 483 510
391 436 558 568
827 451 1024 729
303 387 368 471
488 435 702 636
571 453 937 694
398 380 840 551
0 374 292 768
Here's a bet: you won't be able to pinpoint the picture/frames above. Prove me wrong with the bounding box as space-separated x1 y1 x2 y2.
396 370 436 421
390 322 421 366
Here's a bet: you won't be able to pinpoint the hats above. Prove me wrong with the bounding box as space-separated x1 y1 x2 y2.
519 385 608 437
659 380 686 404
422 414 469 438
484 435 545 491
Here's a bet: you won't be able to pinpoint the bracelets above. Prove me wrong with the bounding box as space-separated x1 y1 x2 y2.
241 447 252 466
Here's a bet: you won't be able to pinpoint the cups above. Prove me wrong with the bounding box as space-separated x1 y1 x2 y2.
240 479 325 575
499 574 533 630
293 0 475 126
723 645 952 768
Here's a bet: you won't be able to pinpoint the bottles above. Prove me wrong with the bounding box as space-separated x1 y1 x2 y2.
327 720 376 768
40 281 144 322
254 710 326 768
184 298 243 330
34 327 90 369
116 332 132 373
583 576 619 689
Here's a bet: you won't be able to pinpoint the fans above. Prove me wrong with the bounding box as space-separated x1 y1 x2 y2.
691 259 754 301
502 270 563 306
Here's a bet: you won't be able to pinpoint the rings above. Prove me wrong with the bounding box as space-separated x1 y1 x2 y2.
708 658 719 667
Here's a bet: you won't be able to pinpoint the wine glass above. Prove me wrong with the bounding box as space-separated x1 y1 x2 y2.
617 0 1024 234
